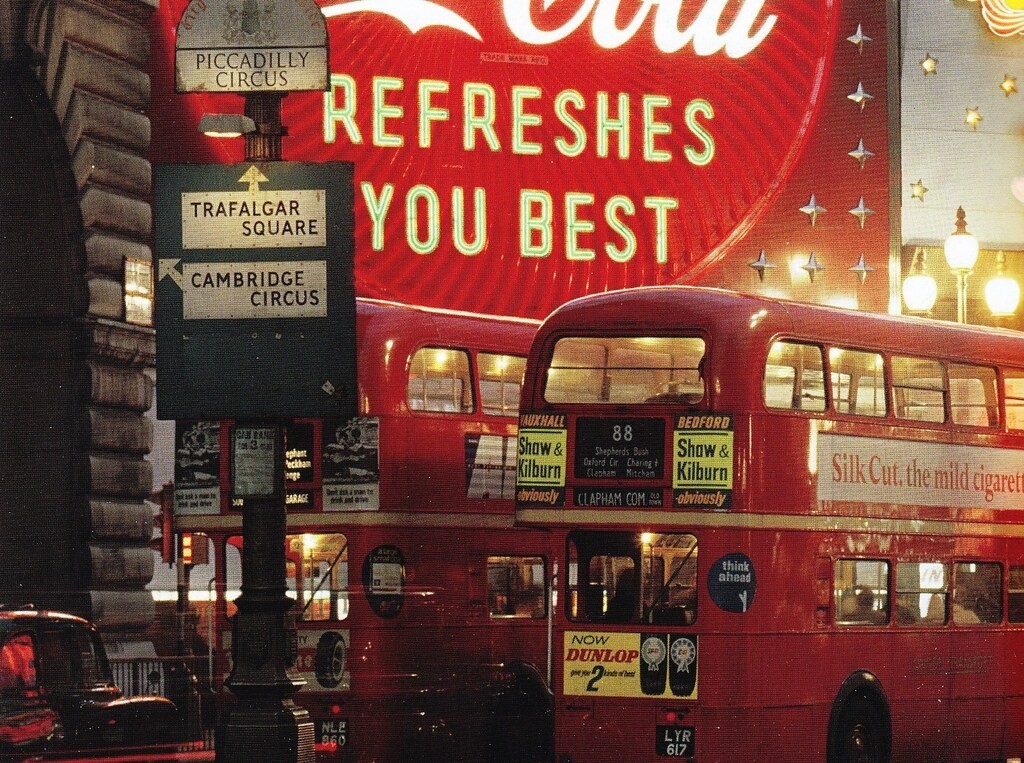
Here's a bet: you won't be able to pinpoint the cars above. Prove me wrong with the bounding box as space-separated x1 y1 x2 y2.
0 608 183 763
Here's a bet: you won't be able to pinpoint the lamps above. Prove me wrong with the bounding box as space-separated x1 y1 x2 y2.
197 110 256 142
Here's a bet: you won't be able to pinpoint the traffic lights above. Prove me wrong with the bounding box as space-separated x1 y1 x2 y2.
146 483 175 564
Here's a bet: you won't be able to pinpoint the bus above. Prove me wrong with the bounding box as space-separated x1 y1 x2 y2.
174 284 1023 763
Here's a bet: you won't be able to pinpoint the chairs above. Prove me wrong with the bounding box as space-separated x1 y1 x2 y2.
841 585 981 623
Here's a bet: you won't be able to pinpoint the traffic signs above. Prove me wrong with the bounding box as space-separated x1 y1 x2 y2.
152 159 362 422
